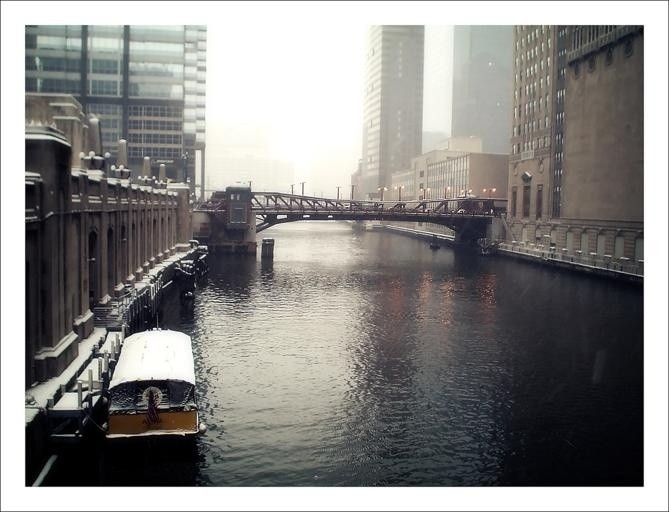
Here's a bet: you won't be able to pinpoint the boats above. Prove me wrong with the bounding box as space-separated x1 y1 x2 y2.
102 327 206 445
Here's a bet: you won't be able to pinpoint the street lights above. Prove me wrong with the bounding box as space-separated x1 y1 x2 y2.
79 148 112 172
156 179 167 187
136 174 156 186
109 162 132 180
180 152 192 184
242 173 502 200
